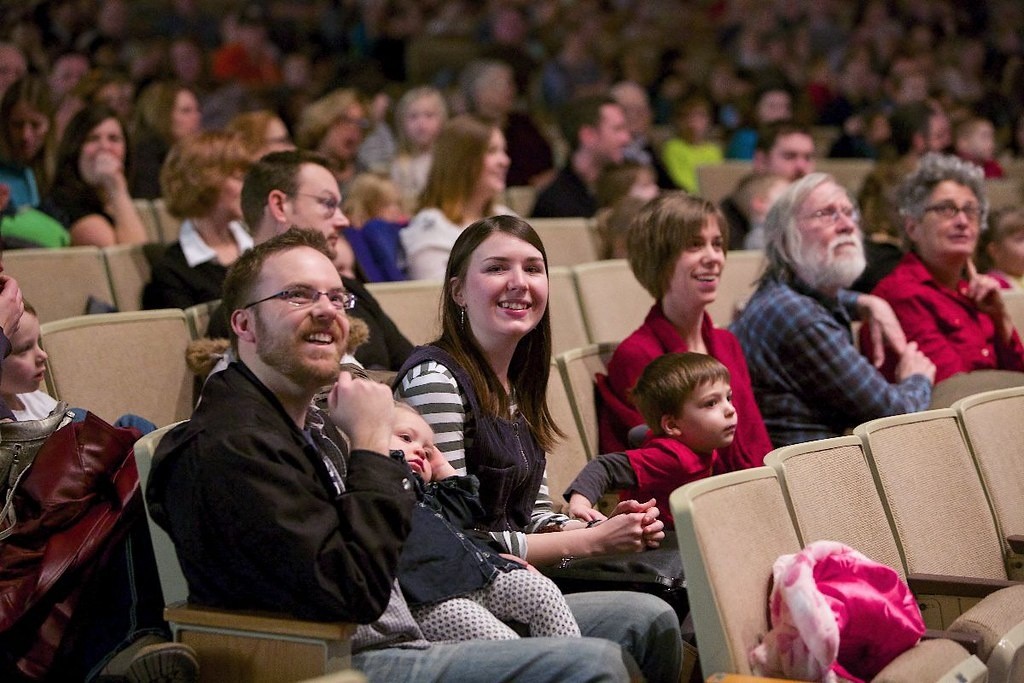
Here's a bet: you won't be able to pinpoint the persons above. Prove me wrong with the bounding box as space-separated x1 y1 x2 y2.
0 0 1024 683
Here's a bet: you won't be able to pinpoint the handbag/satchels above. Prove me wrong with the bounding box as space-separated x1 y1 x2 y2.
0 400 72 500
538 526 686 597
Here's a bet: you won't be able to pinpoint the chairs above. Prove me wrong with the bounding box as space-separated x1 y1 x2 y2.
0 131 1024 683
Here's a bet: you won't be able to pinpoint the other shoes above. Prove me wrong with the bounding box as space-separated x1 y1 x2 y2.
118 637 202 683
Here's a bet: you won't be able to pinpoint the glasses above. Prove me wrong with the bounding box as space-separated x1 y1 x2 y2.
242 285 357 309
288 190 339 217
795 208 857 225
924 200 983 220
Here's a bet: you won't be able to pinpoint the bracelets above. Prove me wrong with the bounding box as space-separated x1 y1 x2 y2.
586 519 602 528
